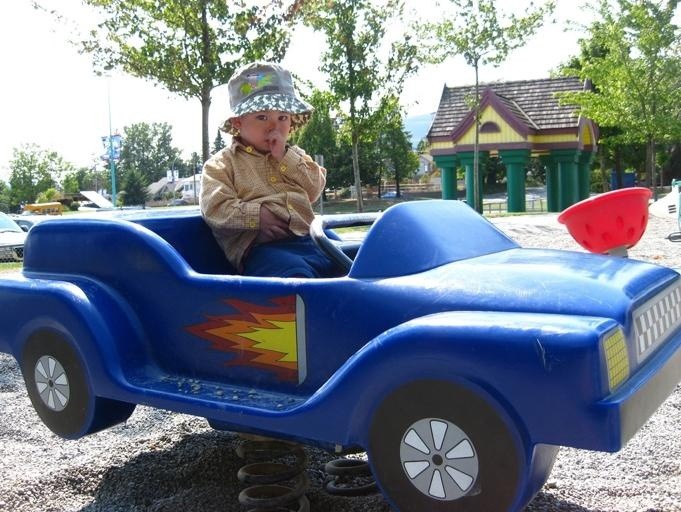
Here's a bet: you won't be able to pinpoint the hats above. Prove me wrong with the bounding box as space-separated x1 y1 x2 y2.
218 62 315 136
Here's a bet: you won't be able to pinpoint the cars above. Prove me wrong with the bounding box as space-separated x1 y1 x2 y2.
380 191 403 198
0 211 58 262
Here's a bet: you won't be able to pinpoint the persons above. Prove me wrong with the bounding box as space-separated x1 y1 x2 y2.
197 60 356 279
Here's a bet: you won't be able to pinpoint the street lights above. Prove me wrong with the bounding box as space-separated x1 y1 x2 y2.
172 148 185 206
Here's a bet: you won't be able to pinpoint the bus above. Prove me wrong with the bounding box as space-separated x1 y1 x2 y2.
23 202 62 215
